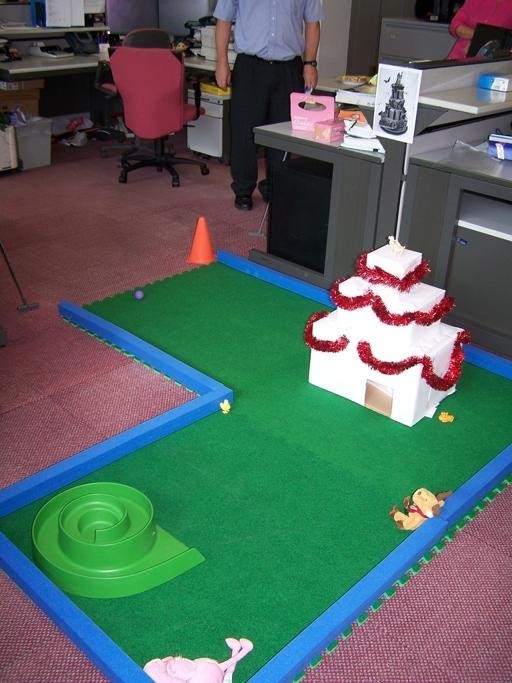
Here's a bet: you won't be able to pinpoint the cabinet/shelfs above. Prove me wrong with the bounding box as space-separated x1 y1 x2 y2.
400 58 510 360
245 58 411 294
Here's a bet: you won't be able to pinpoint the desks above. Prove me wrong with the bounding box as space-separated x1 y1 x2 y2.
0 44 238 168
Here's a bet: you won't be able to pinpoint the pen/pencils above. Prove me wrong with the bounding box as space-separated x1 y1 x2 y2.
348 121 358 131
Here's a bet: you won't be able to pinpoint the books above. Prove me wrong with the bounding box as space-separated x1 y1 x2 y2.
199 26 240 62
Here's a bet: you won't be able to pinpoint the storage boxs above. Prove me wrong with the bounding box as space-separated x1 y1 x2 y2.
10 114 53 173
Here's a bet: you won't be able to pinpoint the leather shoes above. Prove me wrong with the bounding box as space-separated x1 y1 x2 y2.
234 194 254 211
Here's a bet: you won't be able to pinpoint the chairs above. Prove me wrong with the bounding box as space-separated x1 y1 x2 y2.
91 27 211 191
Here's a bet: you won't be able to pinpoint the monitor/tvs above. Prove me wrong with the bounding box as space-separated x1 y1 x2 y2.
105 0 159 36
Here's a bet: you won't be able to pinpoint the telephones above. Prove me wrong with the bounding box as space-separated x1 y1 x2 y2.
63 31 99 55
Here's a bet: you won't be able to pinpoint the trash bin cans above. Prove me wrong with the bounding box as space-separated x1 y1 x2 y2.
13 117 54 171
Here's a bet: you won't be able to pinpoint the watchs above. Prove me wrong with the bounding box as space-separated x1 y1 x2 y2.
303 60 318 65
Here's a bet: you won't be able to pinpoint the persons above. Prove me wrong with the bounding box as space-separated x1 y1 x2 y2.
445 0 512 60
415 0 464 24
212 0 323 211
